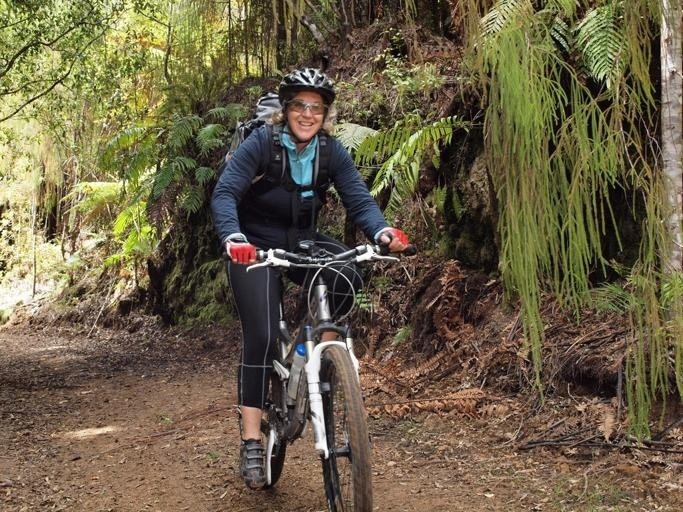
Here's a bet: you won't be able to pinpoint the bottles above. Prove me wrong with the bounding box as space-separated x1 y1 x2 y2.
286 343 307 401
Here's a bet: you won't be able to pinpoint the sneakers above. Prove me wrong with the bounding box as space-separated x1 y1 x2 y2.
240 433 266 489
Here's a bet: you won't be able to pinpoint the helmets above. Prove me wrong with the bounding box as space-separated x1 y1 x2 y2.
278 67 335 106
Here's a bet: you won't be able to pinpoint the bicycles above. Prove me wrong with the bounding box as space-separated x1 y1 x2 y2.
222 234 419 512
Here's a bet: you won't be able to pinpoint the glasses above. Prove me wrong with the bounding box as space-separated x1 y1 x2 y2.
286 98 329 115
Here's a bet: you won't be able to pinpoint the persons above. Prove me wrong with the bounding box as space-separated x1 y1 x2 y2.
209 67 409 489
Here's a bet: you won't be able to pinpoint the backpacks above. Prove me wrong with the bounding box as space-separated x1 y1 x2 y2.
204 93 329 201
